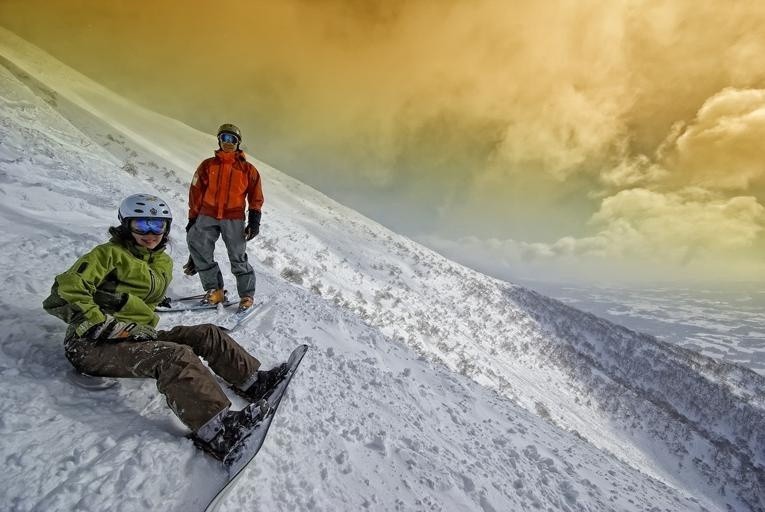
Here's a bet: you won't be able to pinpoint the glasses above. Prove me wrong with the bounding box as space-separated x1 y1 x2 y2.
128 218 168 236
219 134 238 145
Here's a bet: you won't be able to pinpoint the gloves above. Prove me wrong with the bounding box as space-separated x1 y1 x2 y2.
92 314 158 343
185 217 196 232
245 208 261 242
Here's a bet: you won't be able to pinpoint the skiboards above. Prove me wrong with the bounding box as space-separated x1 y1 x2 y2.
155 301 262 334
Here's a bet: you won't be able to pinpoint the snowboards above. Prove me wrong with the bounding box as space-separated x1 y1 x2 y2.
205 346 307 512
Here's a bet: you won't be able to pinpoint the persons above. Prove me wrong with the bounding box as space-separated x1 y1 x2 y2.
42 192 287 464
178 122 265 311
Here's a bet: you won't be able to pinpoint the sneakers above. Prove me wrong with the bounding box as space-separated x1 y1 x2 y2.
200 287 224 305
202 397 271 469
239 295 253 309
228 360 290 405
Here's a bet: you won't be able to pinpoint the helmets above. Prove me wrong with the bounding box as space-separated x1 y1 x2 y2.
117 193 173 238
216 124 242 142
